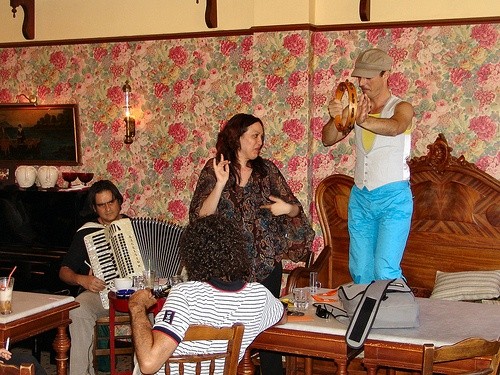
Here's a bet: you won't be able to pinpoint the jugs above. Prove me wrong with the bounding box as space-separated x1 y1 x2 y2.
15 166 37 187
37 166 59 188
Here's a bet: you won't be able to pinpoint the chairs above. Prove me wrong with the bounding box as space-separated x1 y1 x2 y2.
0 310 245 375
421 337 500 375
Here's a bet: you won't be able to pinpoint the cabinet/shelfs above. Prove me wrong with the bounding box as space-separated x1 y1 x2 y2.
0 184 98 365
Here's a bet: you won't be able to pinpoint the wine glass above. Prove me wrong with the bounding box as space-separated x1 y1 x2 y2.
62 172 77 189
78 173 94 186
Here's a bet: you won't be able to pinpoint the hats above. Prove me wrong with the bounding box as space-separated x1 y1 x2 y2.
351 49 394 78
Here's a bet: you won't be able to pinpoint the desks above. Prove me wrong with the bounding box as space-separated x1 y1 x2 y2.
108 291 165 375
238 286 500 375
0 291 81 375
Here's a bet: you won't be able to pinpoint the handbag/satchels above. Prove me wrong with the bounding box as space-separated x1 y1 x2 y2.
339 277 418 331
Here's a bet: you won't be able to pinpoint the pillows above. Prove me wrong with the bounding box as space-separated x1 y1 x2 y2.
430 270 500 300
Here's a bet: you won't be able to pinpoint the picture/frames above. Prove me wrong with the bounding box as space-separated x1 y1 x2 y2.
0 103 79 166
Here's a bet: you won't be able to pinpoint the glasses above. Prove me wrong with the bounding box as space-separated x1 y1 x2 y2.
316 305 334 319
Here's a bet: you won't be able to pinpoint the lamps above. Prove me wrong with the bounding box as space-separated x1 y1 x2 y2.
121 80 135 146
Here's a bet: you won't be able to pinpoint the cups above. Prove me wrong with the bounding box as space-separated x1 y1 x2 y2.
292 288 311 303
110 278 133 289
0 277 14 315
310 272 318 294
142 270 156 295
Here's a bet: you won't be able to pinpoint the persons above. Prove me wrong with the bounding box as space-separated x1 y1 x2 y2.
59 180 287 375
323 49 415 284
188 113 318 375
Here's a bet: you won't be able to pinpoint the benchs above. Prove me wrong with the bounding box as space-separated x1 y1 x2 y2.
286 133 500 375
